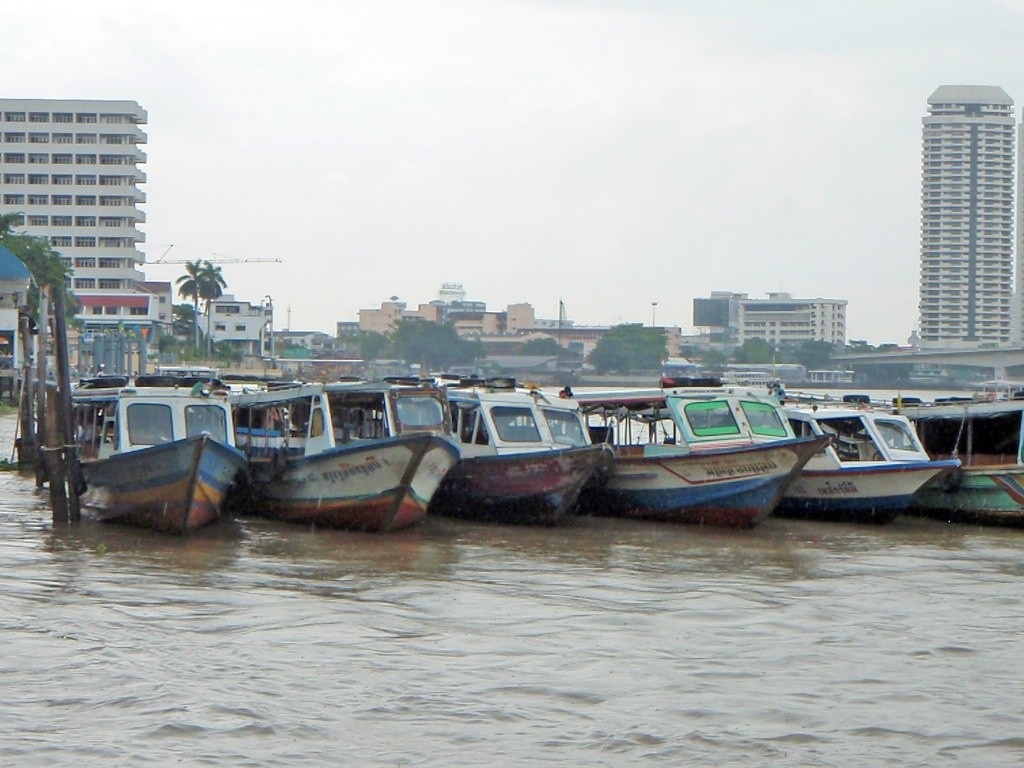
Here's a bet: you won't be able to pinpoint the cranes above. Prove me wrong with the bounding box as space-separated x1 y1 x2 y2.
145 244 283 264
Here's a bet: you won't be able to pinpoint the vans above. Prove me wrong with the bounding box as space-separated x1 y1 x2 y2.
892 397 922 406
844 395 870 403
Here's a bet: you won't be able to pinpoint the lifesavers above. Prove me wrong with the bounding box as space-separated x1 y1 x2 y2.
75 369 517 389
842 394 871 402
893 398 972 407
660 376 723 388
1015 391 1024 400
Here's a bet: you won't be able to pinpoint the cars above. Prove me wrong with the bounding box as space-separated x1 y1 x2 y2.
978 343 998 348
145 353 158 363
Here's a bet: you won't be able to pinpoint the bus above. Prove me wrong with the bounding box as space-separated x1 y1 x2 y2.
808 370 855 383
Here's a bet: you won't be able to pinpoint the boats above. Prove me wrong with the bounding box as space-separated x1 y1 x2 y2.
668 388 718 399
733 372 781 388
236 375 462 531
889 401 1024 521
440 376 616 526
978 381 1009 397
573 386 835 529
71 367 259 402
777 398 962 524
660 363 727 399
81 397 249 534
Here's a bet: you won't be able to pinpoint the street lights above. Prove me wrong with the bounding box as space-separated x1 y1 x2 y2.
652 302 657 326
